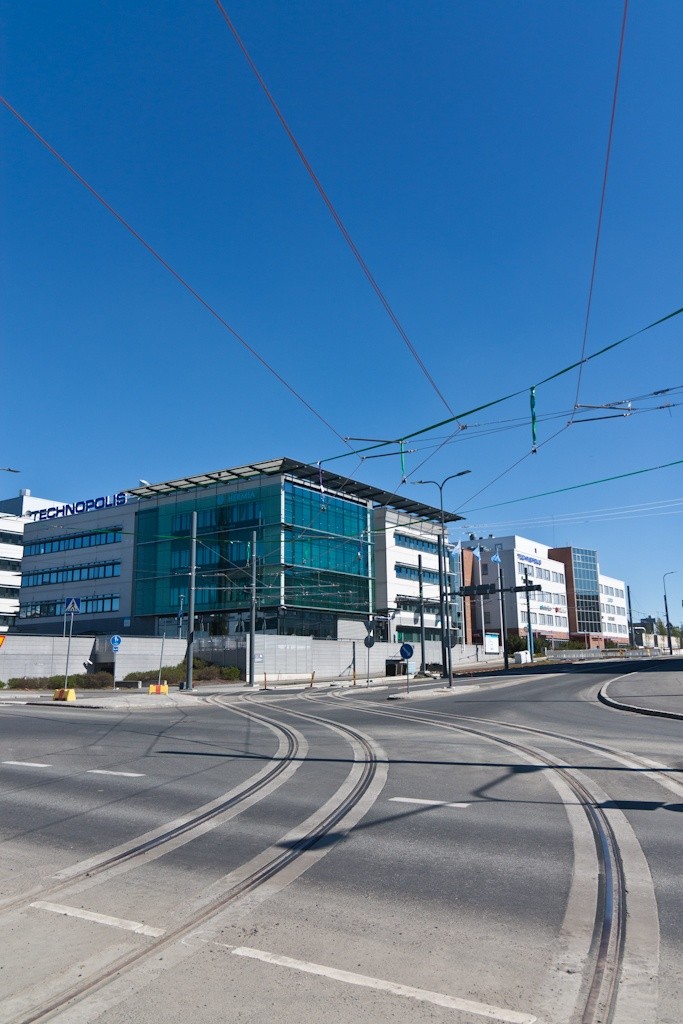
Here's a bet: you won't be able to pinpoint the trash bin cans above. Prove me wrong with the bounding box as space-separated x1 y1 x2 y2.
514 651 532 663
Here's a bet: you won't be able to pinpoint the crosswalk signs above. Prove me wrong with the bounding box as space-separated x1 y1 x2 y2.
65 597 81 614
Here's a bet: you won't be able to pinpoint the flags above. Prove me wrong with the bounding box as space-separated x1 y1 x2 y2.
450 541 461 559
473 546 481 561
491 553 501 563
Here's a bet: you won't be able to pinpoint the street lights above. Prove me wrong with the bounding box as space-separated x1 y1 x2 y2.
409 469 473 677
663 570 678 655
179 595 185 639
